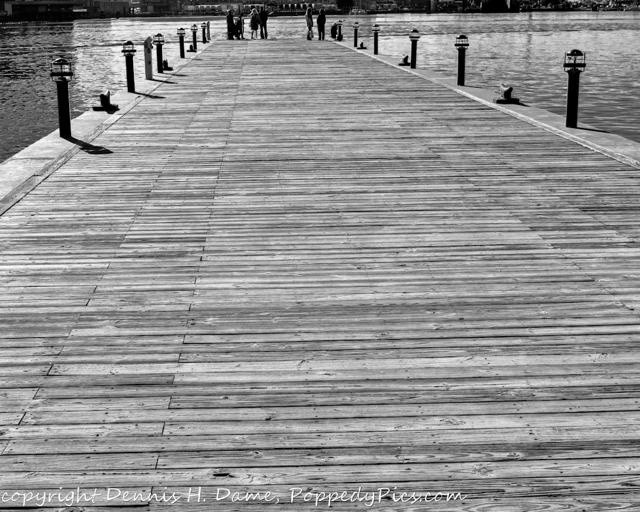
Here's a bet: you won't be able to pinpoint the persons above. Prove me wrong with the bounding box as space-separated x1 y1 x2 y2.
227 10 236 40
331 24 338 39
259 7 269 39
317 8 325 40
236 16 246 40
248 8 260 40
305 7 314 41
